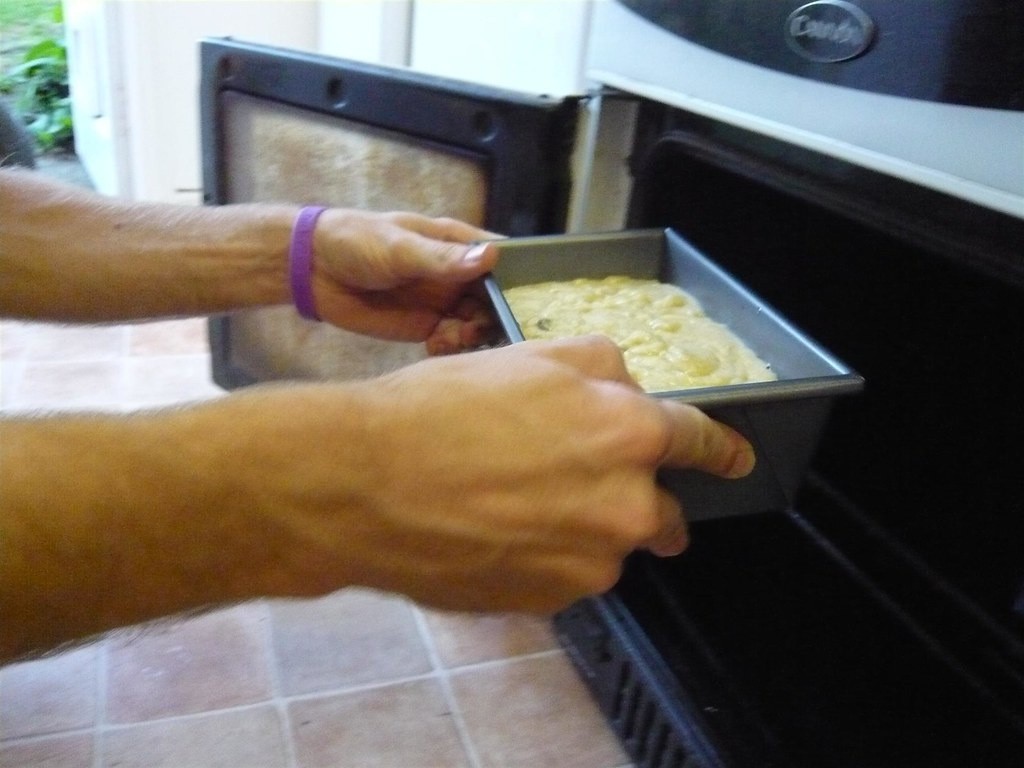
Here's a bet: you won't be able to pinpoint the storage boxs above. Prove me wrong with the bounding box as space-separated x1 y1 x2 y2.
481 226 867 525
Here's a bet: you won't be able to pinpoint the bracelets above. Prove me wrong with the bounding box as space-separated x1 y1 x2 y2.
290 205 326 321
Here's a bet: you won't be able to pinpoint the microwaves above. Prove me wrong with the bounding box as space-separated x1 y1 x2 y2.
198 0 1023 768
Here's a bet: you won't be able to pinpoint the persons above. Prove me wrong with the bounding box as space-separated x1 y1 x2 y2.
1 165 754 672
